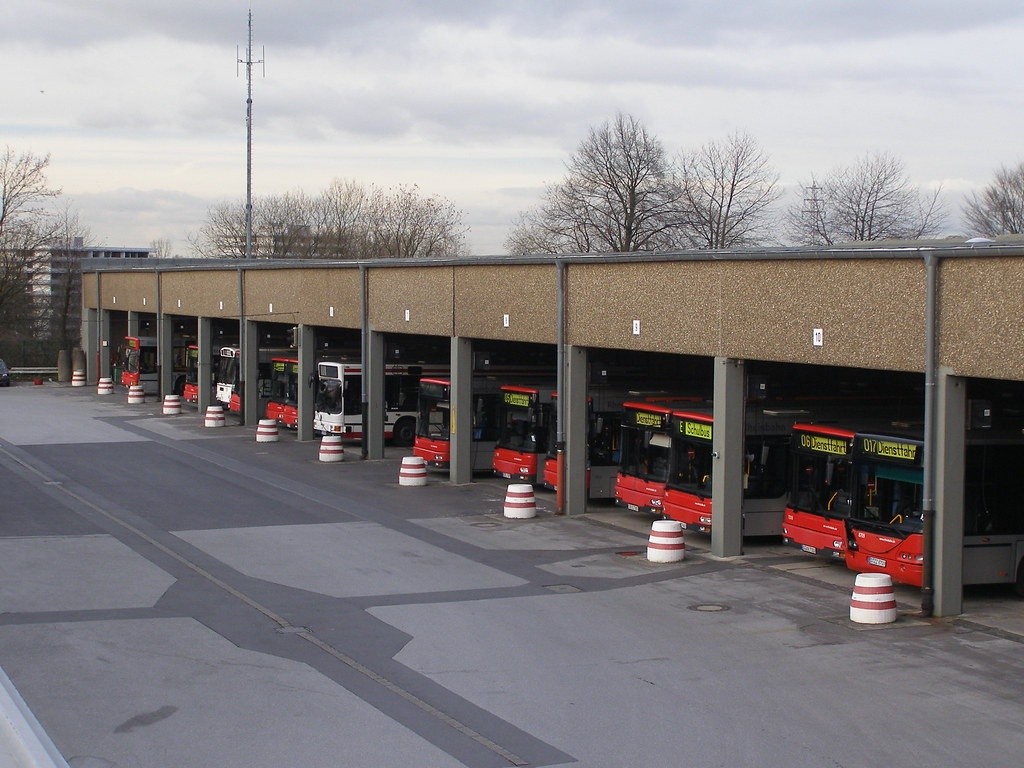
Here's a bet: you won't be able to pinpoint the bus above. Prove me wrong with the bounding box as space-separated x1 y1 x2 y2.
111 334 1024 599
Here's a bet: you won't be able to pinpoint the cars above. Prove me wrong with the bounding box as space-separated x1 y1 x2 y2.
0 358 12 387
